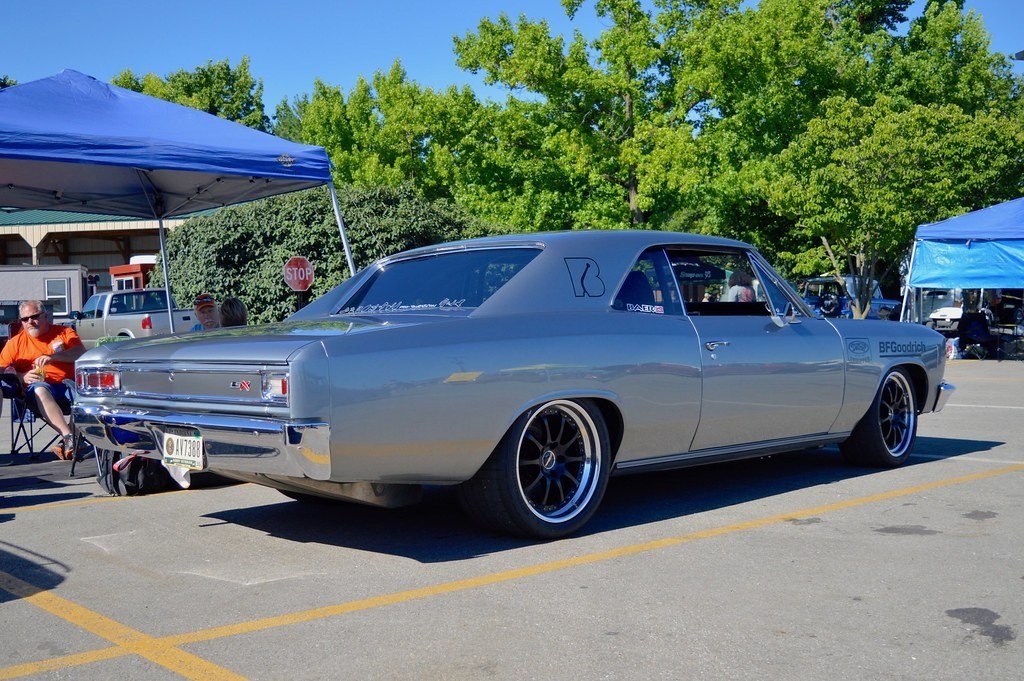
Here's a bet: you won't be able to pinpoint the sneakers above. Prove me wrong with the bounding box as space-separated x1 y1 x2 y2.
53 439 66 461
64 433 86 460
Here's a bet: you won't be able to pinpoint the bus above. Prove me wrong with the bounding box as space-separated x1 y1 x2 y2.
926 288 1024 336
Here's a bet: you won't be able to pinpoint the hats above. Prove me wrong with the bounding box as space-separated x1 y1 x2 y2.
195 293 215 311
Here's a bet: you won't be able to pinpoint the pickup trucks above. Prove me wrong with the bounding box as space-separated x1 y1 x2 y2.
801 276 903 322
70 288 201 352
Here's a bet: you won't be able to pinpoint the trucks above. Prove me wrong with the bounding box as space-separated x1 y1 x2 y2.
0 265 88 333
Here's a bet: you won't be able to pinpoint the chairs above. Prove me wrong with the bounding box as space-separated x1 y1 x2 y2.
621 270 657 314
0 371 76 464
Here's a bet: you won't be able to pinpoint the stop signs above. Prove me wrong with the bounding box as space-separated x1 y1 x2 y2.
283 256 314 292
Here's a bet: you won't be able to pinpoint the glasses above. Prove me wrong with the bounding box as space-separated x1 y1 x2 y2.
193 297 214 305
19 312 44 322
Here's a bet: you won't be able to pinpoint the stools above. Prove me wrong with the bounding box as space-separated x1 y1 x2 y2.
963 341 986 361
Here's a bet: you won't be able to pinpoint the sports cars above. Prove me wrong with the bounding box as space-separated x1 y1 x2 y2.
70 230 957 542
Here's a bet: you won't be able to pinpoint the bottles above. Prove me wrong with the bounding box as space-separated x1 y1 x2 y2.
5 363 16 375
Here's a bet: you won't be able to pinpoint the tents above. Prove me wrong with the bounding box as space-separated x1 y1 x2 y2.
0 68 356 333
896 197 1024 323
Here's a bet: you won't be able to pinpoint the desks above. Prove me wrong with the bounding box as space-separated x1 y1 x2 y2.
989 324 1018 359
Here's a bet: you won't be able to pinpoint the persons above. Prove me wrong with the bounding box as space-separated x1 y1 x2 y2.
189 294 247 334
700 270 755 302
0 299 88 461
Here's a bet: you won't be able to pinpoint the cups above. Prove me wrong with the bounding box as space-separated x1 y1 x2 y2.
32 363 46 381
